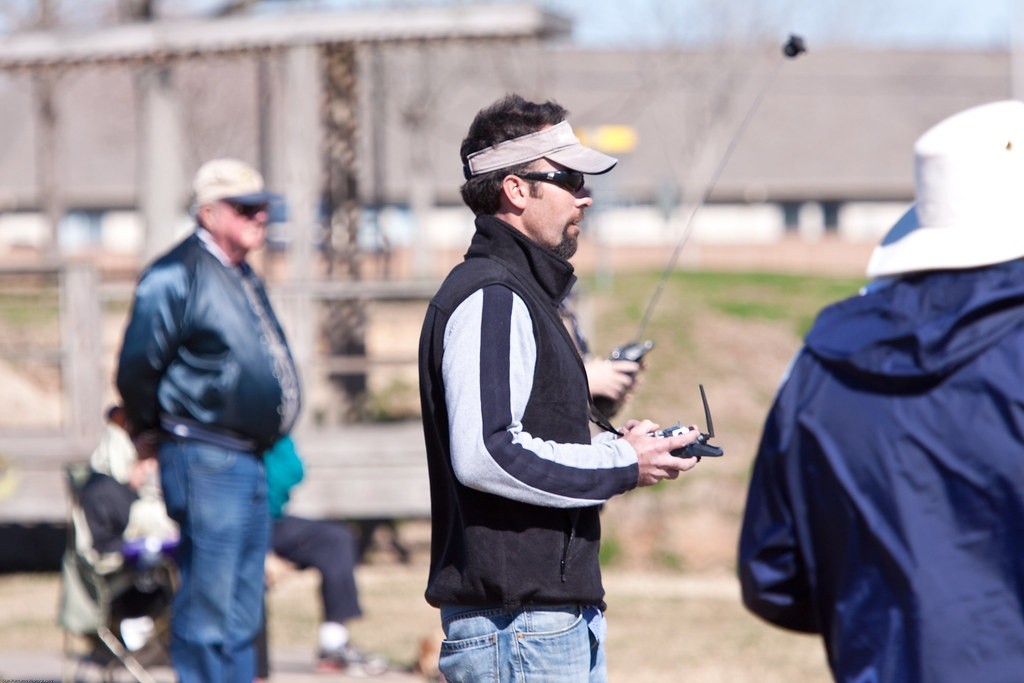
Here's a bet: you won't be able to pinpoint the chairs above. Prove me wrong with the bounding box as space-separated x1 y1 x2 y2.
50 458 181 683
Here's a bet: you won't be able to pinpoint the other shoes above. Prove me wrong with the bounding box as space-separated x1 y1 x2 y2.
317 639 391 677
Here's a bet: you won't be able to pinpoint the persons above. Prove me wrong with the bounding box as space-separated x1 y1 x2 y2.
72 159 385 683
417 97 701 683
737 101 1024 683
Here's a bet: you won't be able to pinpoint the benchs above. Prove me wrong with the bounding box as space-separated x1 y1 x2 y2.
0 420 431 565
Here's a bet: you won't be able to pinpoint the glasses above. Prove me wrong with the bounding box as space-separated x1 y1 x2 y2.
226 200 267 220
515 171 584 194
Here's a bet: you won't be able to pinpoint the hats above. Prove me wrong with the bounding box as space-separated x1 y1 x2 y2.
463 119 619 176
186 159 264 216
866 100 1024 276
